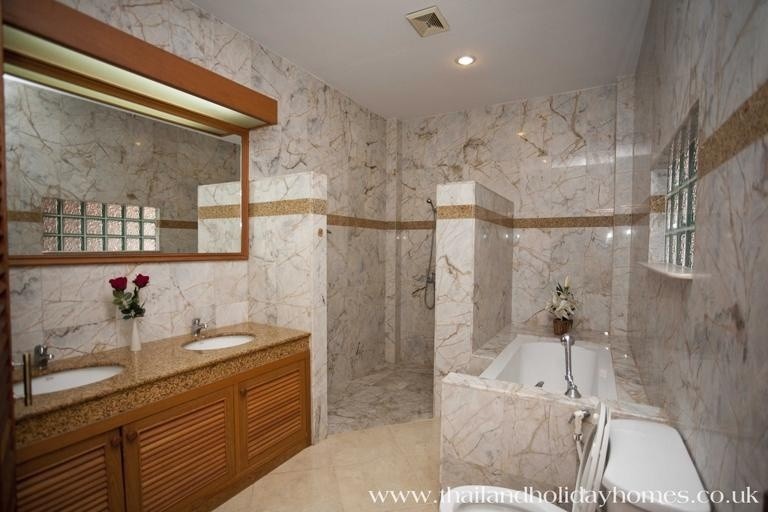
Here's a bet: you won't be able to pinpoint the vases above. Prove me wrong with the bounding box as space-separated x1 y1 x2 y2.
553 318 570 336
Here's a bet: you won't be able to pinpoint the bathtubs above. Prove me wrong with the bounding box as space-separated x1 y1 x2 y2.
478 331 619 402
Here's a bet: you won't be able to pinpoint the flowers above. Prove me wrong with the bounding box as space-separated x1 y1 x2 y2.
545 276 578 319
107 274 148 319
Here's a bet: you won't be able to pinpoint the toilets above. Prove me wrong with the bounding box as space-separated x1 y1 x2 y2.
439 402 712 512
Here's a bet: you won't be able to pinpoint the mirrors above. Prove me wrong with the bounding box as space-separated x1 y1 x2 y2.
5 47 248 261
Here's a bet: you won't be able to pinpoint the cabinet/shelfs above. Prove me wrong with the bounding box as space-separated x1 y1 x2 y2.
237 351 311 493
13 378 237 512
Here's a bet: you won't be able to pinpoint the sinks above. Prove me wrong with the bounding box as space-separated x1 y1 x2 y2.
12 362 127 399
181 331 257 351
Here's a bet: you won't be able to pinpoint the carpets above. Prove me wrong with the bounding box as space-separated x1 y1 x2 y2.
214 420 439 511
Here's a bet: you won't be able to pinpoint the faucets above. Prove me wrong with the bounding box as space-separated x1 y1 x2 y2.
191 317 210 336
32 344 54 368
559 333 582 398
